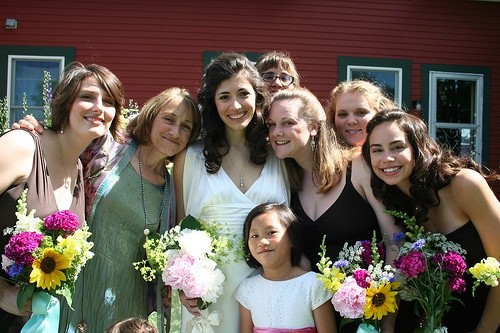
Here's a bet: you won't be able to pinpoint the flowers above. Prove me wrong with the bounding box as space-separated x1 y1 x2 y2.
2 188 96 312
383 209 500 333
316 210 401 333
132 214 251 310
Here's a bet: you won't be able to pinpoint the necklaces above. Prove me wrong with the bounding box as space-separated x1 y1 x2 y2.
138 144 168 236
228 149 251 187
55 131 76 189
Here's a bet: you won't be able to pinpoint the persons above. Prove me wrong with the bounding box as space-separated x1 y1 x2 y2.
11 86 202 333
361 108 500 333
232 202 337 333
325 80 397 150
173 51 311 333
0 61 125 333
261 86 407 333
106 317 158 333
253 51 300 97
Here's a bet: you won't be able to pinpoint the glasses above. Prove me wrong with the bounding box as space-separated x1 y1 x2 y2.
260 72 295 87
